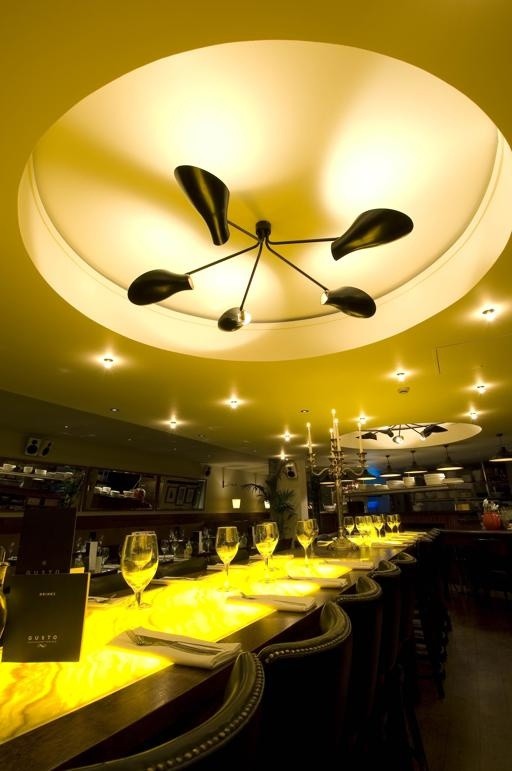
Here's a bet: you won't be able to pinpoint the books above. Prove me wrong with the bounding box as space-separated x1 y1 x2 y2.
2 507 91 662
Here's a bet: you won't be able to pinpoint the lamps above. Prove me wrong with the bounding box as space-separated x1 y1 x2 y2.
320 424 512 484
127 160 416 345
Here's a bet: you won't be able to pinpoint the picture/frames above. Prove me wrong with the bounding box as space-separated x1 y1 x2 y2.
186 488 193 504
165 486 177 506
177 486 186 508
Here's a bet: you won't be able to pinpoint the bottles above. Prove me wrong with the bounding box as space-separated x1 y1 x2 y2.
75 553 83 567
184 542 192 560
86 531 98 572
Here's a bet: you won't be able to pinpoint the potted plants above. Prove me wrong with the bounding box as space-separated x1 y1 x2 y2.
242 459 297 540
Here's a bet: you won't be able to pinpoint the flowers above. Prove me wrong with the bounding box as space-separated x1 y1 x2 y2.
476 497 501 512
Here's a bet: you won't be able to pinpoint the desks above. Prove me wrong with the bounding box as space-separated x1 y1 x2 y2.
437 527 512 602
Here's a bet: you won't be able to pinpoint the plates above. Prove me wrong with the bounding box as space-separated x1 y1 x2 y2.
442 481 464 484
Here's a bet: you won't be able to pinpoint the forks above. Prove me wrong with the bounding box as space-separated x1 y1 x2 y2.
128 630 226 656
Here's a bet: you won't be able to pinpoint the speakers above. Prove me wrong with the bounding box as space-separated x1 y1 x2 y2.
285 463 297 479
38 440 54 457
24 438 40 456
203 465 211 476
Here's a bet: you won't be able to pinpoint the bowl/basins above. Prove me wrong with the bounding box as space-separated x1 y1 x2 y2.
386 473 447 490
359 484 388 491
95 486 135 497
1 462 73 479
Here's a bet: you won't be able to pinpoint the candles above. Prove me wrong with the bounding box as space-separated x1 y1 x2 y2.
302 408 370 453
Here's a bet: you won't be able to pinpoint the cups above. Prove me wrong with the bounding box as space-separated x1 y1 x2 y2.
74 536 84 551
5 542 15 559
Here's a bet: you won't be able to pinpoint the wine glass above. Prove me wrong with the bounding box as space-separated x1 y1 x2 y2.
296 517 323 563
171 541 180 559
161 540 170 560
121 532 157 613
102 548 110 571
215 526 240 585
341 513 404 545
251 523 282 580
206 538 212 553
